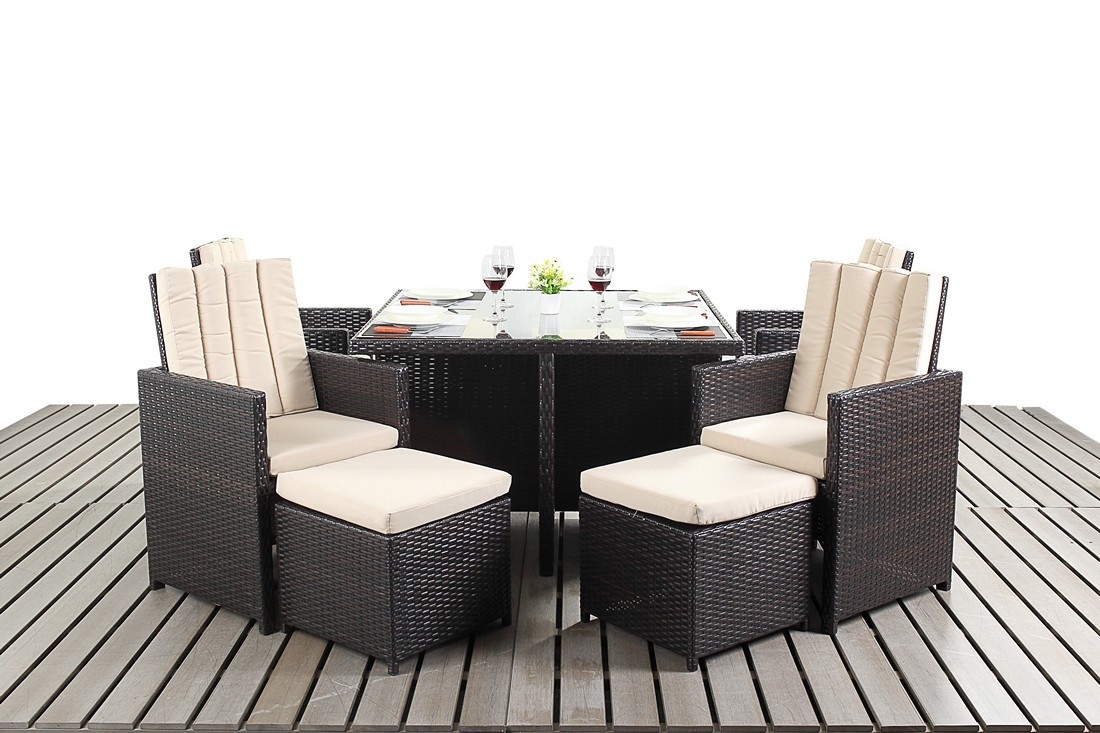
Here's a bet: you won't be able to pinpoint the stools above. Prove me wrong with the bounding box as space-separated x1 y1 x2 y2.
578 443 817 671
273 446 513 675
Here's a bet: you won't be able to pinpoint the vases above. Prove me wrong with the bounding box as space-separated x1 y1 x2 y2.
539 292 561 315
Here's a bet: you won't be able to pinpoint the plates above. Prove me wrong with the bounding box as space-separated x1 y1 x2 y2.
640 305 699 320
403 288 474 300
630 292 699 303
379 305 448 325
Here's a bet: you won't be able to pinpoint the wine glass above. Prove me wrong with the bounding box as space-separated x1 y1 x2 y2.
587 256 612 324
591 246 615 310
490 243 515 309
481 255 508 322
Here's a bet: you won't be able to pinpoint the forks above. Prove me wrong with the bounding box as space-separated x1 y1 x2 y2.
372 326 435 334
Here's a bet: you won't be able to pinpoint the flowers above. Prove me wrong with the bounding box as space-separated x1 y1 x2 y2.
527 257 575 294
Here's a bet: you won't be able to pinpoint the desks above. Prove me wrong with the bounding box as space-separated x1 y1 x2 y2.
347 289 743 575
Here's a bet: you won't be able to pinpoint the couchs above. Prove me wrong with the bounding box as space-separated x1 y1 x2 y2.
736 238 915 358
136 257 415 636
189 236 375 362
689 257 964 635
